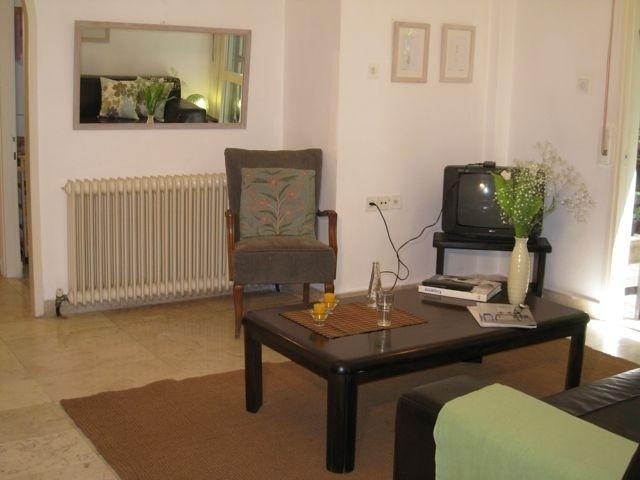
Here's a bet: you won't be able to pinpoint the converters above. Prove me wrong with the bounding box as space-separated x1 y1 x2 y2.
483 161 495 167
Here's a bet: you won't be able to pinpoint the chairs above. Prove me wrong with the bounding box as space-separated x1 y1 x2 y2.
220 144 341 340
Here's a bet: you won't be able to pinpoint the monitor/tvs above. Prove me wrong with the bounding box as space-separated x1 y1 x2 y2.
442 165 545 242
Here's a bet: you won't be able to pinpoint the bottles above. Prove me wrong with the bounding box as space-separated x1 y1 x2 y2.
367 261 383 309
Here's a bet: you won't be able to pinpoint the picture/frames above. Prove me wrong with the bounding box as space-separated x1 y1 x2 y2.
438 22 477 85
389 20 430 84
80 26 110 44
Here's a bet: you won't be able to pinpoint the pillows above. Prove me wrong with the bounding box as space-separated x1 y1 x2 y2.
135 76 174 123
98 76 139 120
236 166 318 241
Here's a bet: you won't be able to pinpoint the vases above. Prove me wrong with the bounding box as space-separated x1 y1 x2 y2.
506 234 532 305
146 113 155 123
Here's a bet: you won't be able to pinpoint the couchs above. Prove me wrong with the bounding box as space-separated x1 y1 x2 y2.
393 365 640 479
80 73 207 123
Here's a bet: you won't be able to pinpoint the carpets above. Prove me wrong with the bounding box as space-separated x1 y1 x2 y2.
60 334 639 478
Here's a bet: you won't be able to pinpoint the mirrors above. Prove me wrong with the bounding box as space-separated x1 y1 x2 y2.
71 20 252 132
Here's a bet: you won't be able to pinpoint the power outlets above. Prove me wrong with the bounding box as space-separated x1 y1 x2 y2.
366 196 377 212
376 195 391 211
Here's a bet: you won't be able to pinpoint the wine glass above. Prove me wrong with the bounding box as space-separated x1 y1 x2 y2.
320 298 340 313
309 310 330 327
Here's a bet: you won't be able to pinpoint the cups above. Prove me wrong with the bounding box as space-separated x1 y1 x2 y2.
376 290 396 327
375 330 392 353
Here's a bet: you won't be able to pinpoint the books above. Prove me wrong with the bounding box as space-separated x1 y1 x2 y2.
418 273 503 303
476 302 538 329
466 305 483 327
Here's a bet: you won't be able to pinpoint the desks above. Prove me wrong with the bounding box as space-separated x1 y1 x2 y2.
432 230 552 298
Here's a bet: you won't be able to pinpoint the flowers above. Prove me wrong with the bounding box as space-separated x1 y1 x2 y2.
136 72 181 113
492 140 593 238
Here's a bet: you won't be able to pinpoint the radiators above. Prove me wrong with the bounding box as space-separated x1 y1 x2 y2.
56 172 274 315
